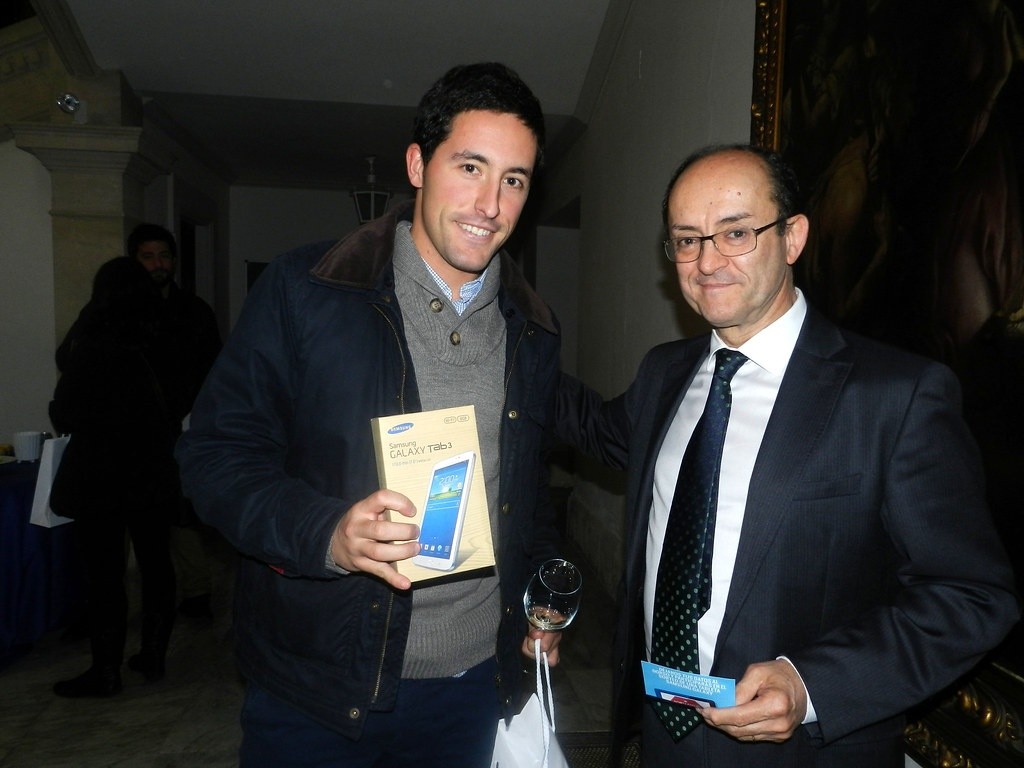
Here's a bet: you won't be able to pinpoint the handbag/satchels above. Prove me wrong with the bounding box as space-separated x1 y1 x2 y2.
491 638 571 767
29 432 75 528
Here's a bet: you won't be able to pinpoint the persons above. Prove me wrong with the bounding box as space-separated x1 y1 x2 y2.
125 218 226 566
48 254 178 697
555 145 1024 768
167 61 594 768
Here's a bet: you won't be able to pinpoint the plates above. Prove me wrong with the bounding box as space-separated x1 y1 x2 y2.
0 455 16 464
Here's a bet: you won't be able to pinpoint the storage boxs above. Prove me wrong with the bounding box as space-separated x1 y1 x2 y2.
370 404 496 584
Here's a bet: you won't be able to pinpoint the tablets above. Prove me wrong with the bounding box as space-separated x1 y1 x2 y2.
413 452 476 571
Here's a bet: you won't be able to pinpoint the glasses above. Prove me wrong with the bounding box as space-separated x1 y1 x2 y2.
663 217 786 263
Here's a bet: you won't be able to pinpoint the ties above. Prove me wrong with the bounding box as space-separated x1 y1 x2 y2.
647 349 747 745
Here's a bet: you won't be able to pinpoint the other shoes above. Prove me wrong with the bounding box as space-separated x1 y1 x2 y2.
53 664 124 699
137 655 167 682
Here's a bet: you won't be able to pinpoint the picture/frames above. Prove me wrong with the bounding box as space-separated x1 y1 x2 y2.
750 0 1024 768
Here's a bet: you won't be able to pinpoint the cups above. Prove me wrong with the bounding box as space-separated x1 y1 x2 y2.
41 431 53 448
12 430 41 461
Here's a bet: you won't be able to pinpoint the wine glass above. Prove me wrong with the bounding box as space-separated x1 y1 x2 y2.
522 559 583 674
